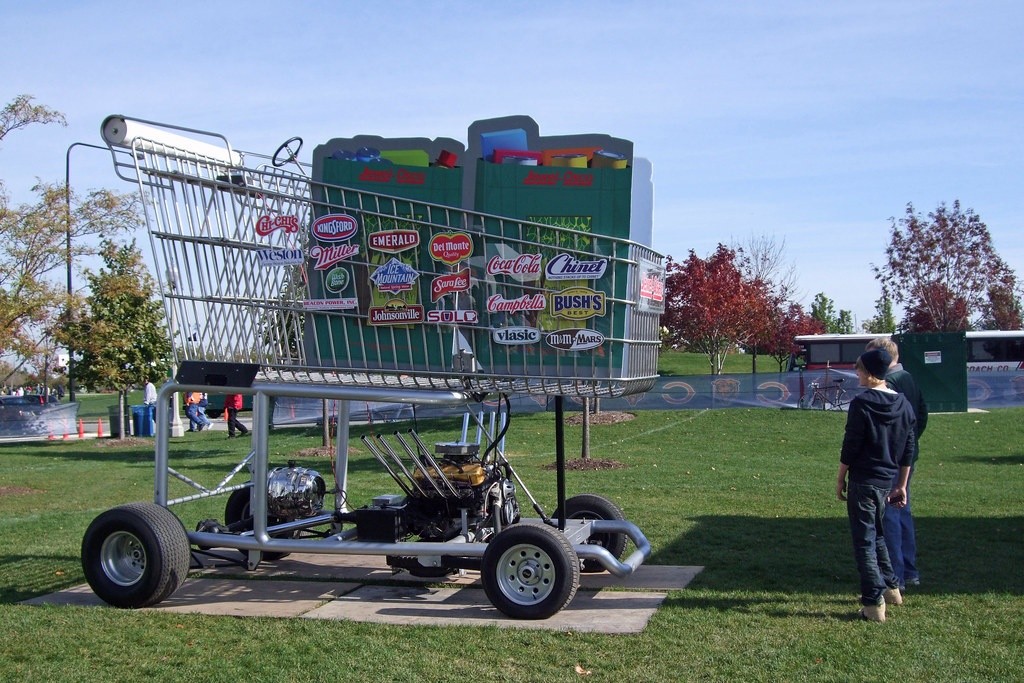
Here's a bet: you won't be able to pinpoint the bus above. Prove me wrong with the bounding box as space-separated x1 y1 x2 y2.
780 329 1024 412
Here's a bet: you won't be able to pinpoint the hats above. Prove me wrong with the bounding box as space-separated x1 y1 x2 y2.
861 348 892 380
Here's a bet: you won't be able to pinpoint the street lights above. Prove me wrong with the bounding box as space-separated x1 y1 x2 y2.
66 142 144 403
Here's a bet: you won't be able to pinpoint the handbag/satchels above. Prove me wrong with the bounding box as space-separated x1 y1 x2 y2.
224 408 229 421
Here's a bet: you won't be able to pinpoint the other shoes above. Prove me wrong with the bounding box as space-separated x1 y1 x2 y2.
899 585 906 590
858 595 886 623
883 588 903 605
185 429 195 432
207 422 214 429
225 436 236 440
241 430 248 437
199 423 206 431
906 576 921 586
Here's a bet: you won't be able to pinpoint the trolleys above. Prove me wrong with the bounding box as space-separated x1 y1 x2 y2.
82 114 665 622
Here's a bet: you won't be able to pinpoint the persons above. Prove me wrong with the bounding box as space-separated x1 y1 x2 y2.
140 376 157 423
0 383 65 400
864 337 928 591
835 349 917 623
182 391 214 432
222 393 252 441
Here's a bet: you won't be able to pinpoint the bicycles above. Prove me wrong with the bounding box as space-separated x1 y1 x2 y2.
799 378 861 414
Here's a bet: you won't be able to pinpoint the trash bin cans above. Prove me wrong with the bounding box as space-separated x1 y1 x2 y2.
131 405 155 437
108 404 132 438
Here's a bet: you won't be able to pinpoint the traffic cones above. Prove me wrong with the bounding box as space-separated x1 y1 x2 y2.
78 419 85 440
46 419 56 441
59 417 70 441
97 417 104 438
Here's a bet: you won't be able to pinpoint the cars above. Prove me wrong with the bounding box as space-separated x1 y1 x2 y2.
0 393 67 438
